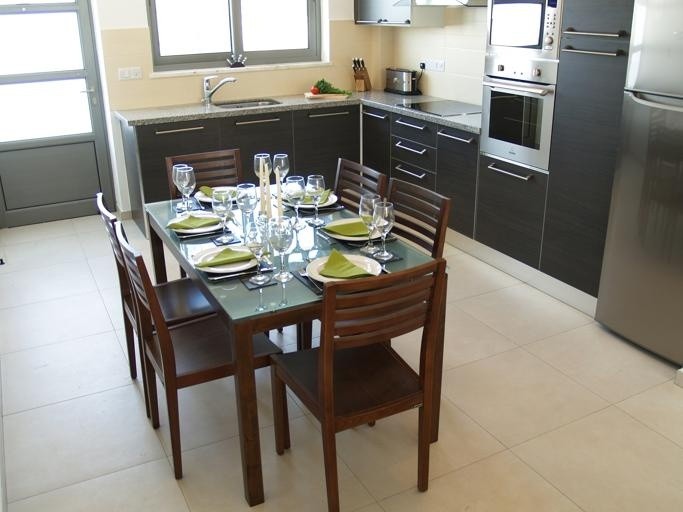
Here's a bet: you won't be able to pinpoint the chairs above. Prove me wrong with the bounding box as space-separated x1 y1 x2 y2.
301 176 450 347
115 221 291 480
96 192 216 417
166 149 246 279
278 157 387 350
269 258 447 511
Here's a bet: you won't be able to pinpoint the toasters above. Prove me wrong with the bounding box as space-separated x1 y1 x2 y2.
385 68 421 94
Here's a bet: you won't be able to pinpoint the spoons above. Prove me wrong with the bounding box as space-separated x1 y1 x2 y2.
347 236 395 248
298 205 344 215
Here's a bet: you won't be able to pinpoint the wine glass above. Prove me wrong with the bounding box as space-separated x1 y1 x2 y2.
252 287 267 312
284 176 306 230
306 175 325 227
254 154 272 200
359 194 380 255
373 202 395 261
276 283 290 307
273 153 288 197
172 164 294 286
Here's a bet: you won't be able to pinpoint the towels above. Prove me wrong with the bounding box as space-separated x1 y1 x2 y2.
288 188 332 205
199 186 239 199
165 214 228 228
319 218 371 238
319 248 377 278
193 246 271 269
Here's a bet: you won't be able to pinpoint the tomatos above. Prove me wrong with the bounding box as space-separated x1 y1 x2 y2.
310 85 320 95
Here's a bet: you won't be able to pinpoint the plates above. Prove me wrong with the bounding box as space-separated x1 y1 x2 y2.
281 191 337 209
168 216 222 234
327 218 382 241
306 254 382 281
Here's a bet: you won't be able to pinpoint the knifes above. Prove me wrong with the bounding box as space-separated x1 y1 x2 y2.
351 58 364 74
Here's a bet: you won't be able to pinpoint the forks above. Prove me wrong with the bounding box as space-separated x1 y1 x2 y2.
296 267 323 292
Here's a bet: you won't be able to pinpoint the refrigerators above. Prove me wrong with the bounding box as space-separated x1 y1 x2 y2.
590 1 681 369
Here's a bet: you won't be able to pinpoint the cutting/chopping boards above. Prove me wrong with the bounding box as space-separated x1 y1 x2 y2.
303 92 347 99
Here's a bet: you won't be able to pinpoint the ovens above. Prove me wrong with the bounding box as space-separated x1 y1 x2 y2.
478 1 564 173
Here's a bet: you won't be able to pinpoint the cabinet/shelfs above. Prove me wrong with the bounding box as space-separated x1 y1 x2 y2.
472 152 549 272
388 110 435 218
538 0 632 298
295 104 361 194
435 121 478 240
222 112 294 187
121 110 223 237
361 103 390 193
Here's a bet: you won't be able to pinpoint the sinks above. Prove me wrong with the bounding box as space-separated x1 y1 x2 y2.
213 99 281 109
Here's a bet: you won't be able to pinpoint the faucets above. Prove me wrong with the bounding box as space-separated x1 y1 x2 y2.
203 75 237 103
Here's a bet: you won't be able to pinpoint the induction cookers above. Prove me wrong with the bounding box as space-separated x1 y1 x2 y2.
397 99 482 118
